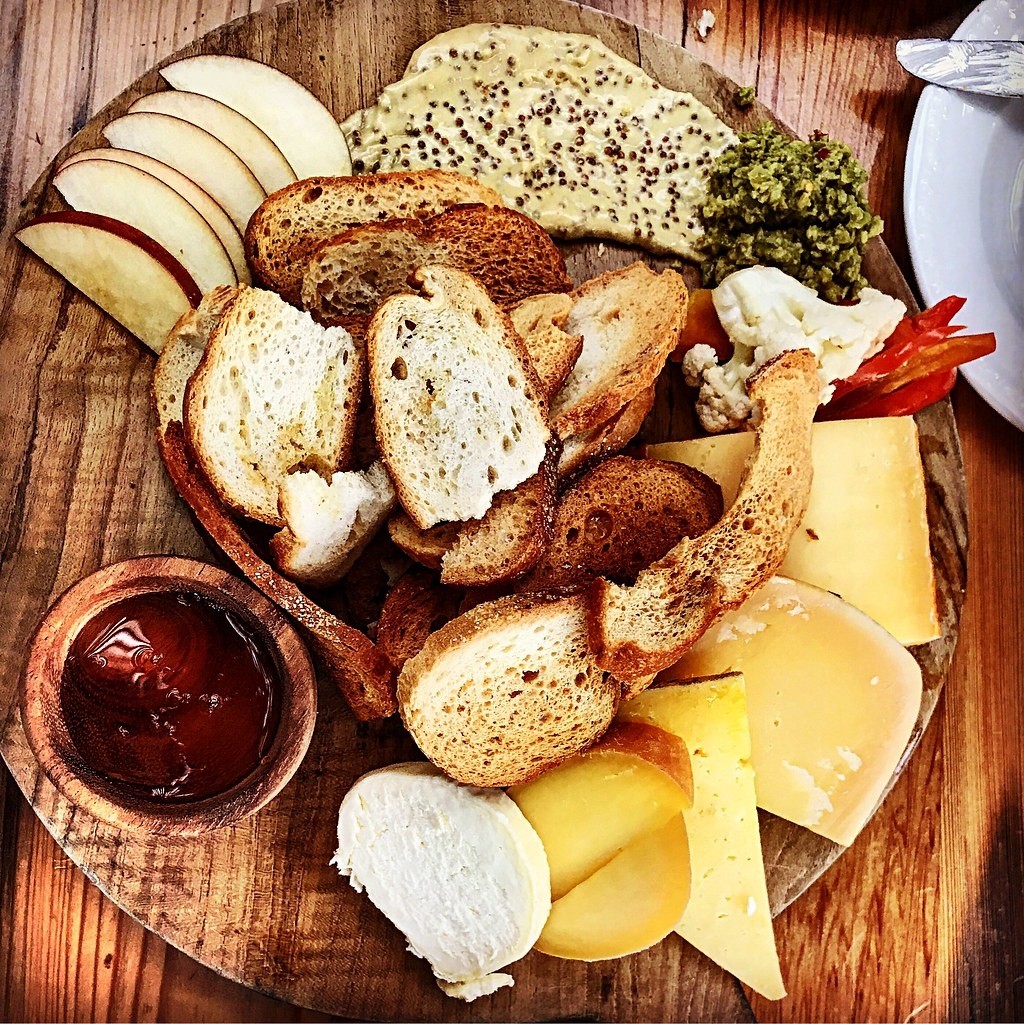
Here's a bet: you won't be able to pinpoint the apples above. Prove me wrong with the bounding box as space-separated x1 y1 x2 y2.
15 56 351 351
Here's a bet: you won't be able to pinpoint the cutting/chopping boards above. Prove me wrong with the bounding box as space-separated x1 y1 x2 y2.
0 0 970 1020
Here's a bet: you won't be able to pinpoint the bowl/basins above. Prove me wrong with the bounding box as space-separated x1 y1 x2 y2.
21 555 322 842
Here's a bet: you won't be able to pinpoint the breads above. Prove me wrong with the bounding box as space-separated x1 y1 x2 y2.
154 172 823 1002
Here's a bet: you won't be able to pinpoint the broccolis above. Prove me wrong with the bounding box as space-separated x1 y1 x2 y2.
681 259 900 431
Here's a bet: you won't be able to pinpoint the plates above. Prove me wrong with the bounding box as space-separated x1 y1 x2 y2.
904 1 1024 439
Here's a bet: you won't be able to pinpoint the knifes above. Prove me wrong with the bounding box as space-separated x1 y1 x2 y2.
896 38 1024 98
